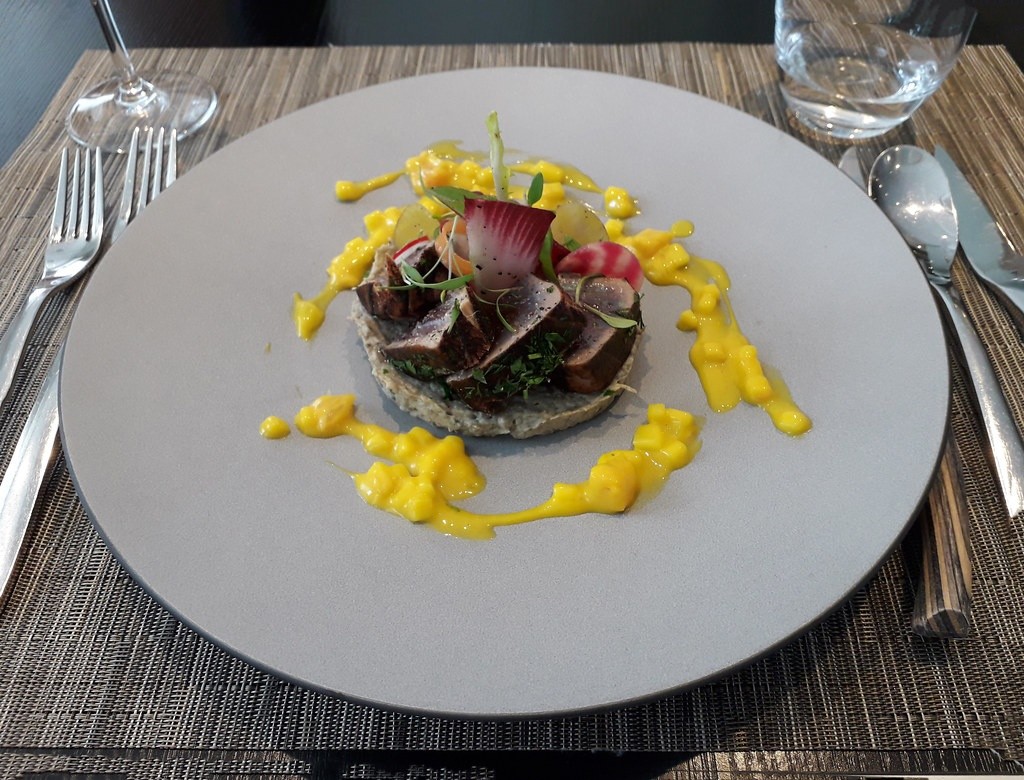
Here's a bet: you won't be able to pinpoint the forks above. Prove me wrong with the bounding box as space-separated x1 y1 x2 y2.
0 127 176 602
0 145 104 410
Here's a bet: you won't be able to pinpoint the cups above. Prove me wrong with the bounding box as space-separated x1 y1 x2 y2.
774 0 978 141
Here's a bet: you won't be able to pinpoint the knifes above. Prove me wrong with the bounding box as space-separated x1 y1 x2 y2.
838 144 973 638
934 144 1024 314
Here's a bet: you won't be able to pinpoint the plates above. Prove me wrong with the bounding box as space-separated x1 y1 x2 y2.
56 66 949 720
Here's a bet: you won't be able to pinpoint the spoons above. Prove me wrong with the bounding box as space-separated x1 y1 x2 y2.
867 145 1024 519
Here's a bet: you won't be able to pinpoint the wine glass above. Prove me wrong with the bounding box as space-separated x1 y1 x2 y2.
64 0 217 152
